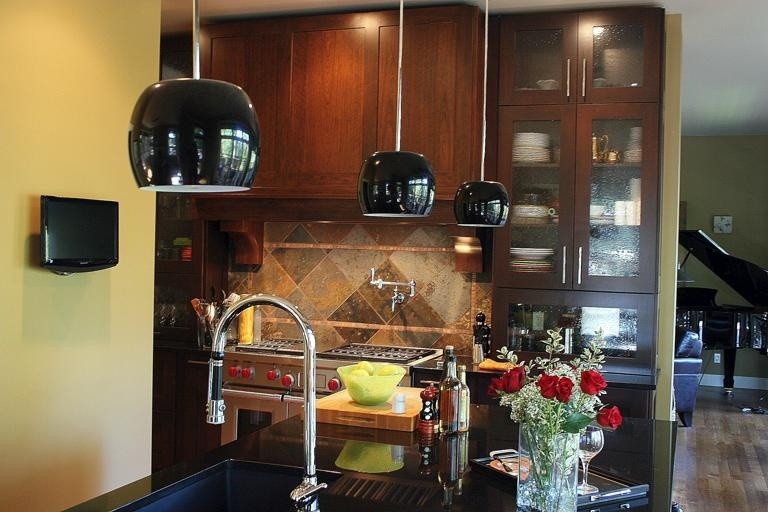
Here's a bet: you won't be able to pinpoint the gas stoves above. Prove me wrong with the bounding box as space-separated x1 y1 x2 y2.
221 336 446 361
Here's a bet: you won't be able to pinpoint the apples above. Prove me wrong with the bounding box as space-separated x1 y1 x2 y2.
349 361 402 377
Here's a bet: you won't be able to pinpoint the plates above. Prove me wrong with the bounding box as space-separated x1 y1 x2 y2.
623 125 642 163
513 130 553 163
509 247 556 272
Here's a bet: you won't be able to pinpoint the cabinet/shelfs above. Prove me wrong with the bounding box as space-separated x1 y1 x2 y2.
496 9 663 105
488 288 658 365
493 106 663 295
200 14 486 205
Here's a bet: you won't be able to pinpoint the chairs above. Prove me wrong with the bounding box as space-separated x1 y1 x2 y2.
673 328 703 427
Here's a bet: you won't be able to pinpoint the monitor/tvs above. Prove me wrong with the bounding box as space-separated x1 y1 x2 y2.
39 193 120 276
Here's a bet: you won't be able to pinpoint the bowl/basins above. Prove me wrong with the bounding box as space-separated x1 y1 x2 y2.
335 361 407 407
333 440 405 475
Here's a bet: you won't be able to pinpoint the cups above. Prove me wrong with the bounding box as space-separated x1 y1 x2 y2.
590 205 609 217
390 443 403 464
471 343 484 364
391 393 407 415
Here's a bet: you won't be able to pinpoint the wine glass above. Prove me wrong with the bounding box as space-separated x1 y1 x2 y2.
576 425 604 495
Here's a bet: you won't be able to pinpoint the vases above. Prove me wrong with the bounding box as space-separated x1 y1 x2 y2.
516 420 580 512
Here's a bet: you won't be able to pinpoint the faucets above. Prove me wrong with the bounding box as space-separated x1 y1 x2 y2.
391 282 407 313
205 292 317 475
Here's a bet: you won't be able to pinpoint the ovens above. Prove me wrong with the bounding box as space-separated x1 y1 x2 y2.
220 360 353 454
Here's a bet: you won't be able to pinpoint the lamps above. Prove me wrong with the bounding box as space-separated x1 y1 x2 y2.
452 1 513 231
354 4 439 222
125 0 260 195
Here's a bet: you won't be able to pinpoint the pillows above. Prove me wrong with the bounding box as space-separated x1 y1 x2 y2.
675 325 699 357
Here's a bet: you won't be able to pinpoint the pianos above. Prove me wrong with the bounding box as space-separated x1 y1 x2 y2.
676 229 767 396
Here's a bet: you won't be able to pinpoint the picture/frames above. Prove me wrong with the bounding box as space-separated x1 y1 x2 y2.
713 214 734 234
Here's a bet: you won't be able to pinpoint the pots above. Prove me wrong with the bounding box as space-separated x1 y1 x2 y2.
515 187 550 204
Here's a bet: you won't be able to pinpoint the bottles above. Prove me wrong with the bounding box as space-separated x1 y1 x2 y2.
471 311 490 354
438 342 471 435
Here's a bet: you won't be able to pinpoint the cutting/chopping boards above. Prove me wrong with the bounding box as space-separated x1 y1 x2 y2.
299 379 428 434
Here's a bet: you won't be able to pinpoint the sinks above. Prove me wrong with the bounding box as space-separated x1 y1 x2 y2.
113 458 343 512
